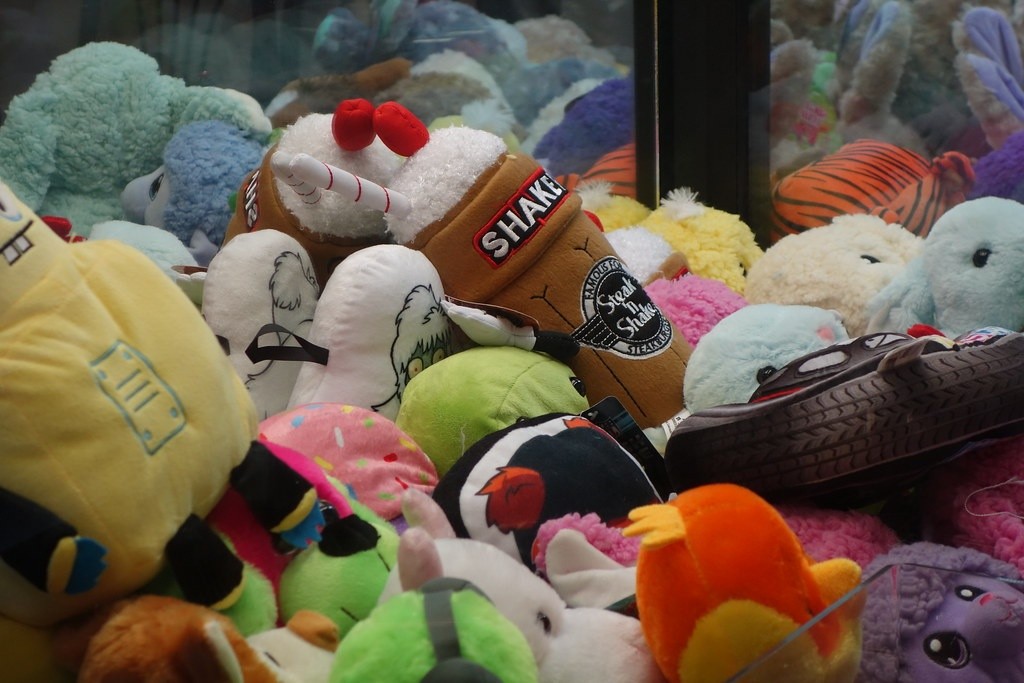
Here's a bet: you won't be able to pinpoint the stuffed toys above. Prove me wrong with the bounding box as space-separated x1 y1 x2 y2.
288 98 694 433
0 1 1024 683
899 196 1023 344
200 226 320 427
682 302 851 411
289 243 579 424
381 489 664 683
75 594 337 683
391 345 590 480
622 482 863 683
327 575 539 681
0 173 327 611
862 538 1024 681
